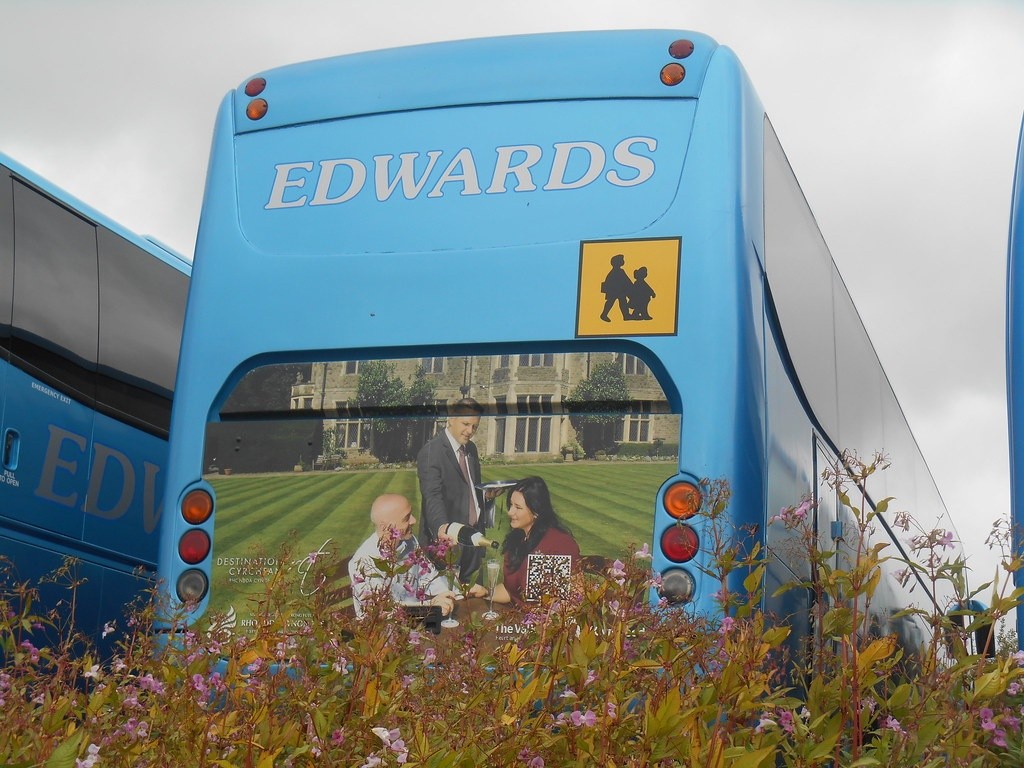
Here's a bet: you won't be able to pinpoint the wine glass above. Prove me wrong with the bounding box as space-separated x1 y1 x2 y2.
440 565 463 629
481 560 502 623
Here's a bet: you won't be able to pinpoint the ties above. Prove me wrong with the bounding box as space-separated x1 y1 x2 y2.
457 447 477 527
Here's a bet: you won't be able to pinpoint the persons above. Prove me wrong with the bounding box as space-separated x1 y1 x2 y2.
461 476 584 622
415 398 500 596
347 494 457 625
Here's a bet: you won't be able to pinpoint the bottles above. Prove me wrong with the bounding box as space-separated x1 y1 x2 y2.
443 520 501 550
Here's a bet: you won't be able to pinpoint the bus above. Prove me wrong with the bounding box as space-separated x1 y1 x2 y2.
151 30 996 768
0 152 193 725
